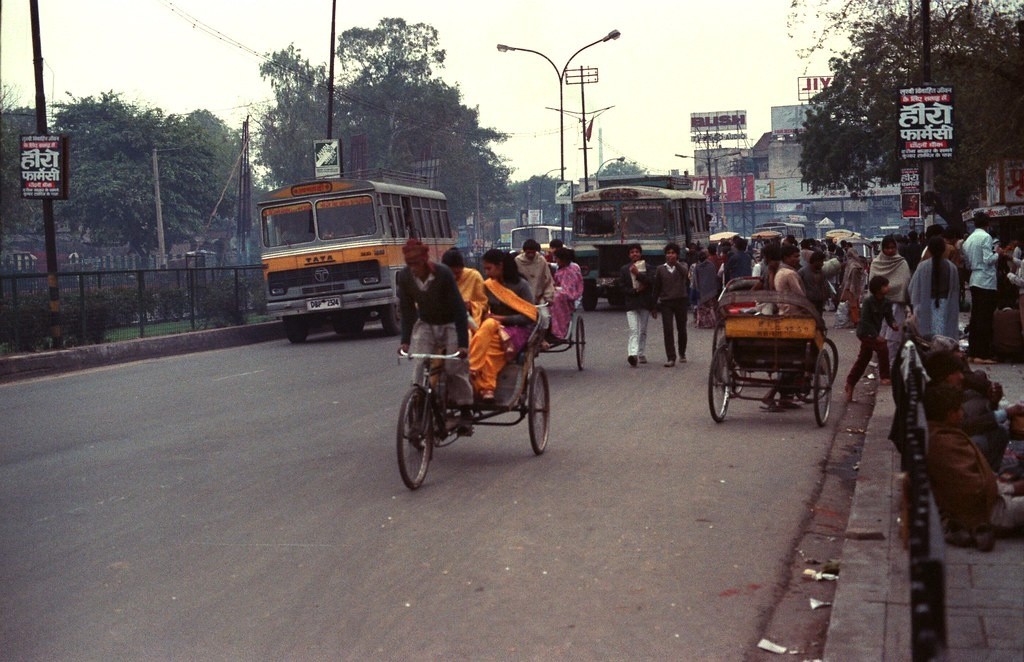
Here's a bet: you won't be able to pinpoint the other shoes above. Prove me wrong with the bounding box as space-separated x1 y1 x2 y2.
639 356 647 363
880 379 891 385
460 416 473 432
846 383 854 401
680 357 687 362
664 360 675 366
973 523 995 552
628 355 637 366
944 518 967 542
483 391 494 401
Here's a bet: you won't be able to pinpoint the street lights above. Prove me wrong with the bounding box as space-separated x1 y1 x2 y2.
675 152 741 234
596 157 625 189
476 180 496 249
539 167 567 226
497 29 621 244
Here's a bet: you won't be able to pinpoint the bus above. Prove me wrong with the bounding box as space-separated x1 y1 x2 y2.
256 167 457 343
509 226 573 256
494 219 517 247
572 187 712 312
870 226 900 238
753 222 807 239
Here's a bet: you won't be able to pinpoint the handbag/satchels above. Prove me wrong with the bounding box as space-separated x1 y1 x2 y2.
991 306 1021 357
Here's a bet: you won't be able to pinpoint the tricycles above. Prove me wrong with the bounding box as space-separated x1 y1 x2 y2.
516 263 586 372
707 275 839 427
712 275 764 397
395 304 550 491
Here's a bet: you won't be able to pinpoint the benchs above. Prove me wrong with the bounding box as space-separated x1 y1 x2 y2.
717 276 761 315
515 306 544 361
723 315 825 366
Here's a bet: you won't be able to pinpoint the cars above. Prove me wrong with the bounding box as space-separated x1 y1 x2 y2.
464 252 484 269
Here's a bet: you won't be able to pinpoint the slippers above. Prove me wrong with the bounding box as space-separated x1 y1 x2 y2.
759 401 785 412
778 403 803 409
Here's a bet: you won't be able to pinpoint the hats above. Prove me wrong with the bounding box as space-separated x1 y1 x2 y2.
929 354 962 381
402 240 430 263
932 335 959 353
441 247 464 267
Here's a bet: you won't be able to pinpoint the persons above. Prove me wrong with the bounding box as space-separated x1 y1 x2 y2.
685 235 868 328
842 223 1024 402
762 246 806 411
618 244 691 369
396 238 583 437
902 310 1024 541
